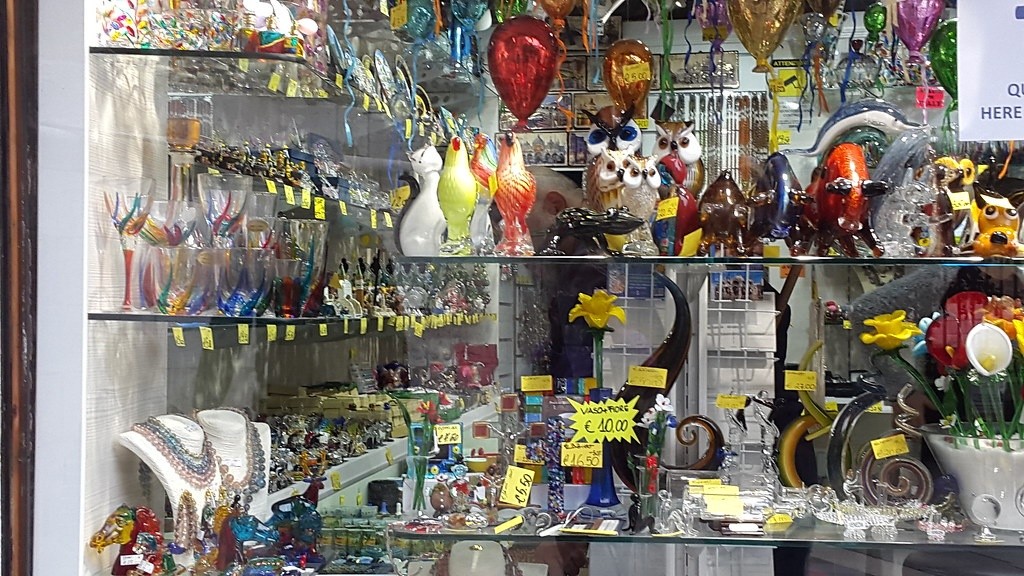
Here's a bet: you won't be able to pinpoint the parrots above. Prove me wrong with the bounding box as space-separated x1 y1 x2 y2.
439 131 538 257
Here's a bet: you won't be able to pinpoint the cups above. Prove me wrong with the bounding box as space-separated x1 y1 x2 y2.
151 247 225 315
216 248 275 318
275 220 325 319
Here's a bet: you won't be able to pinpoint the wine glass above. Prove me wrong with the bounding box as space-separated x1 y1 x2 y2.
91 173 278 319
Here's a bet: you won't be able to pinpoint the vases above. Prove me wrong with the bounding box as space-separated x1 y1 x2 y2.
586 387 622 507
632 455 659 520
403 456 434 522
920 421 1024 530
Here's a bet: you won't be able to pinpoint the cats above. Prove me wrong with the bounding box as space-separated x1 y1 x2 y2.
398 139 447 256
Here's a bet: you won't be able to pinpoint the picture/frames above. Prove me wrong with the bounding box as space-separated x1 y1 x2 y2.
495 95 573 131
666 50 740 91
585 54 661 93
567 130 592 168
504 130 569 169
556 16 624 55
572 93 650 132
548 53 586 92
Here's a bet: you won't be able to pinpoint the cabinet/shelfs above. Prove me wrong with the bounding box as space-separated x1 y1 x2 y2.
83 0 1024 576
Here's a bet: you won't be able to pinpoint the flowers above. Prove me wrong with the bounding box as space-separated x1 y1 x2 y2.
390 392 459 510
859 291 1024 448
637 394 678 468
569 288 627 388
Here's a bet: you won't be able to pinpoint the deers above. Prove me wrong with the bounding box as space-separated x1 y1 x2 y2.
270 446 328 519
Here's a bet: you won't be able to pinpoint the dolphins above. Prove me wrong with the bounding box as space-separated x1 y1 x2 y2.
776 95 935 156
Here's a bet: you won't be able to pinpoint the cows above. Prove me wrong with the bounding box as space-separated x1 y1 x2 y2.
696 145 891 258
